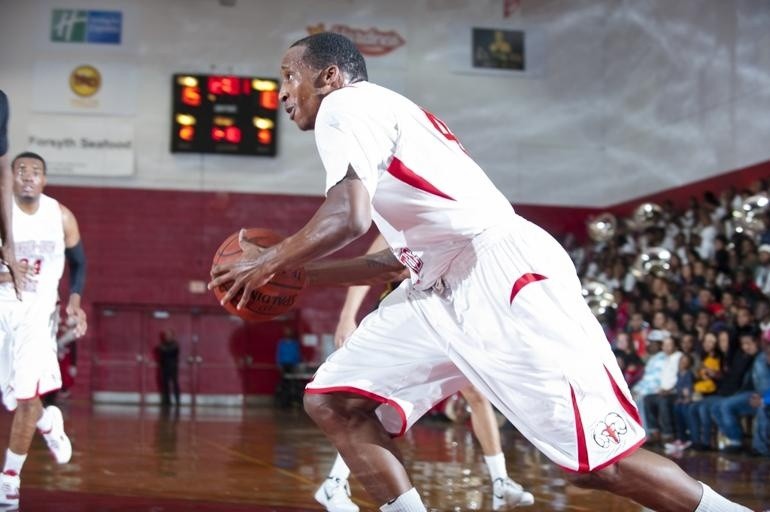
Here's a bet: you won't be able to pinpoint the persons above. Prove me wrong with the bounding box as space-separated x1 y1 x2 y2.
154 329 180 406
561 182 770 456
1 152 87 508
315 231 536 512
206 31 754 511
2 88 27 305
277 327 301 371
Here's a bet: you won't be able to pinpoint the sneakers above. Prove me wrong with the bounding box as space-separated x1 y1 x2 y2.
41 406 72 464
0 470 20 510
314 477 359 512
492 478 534 511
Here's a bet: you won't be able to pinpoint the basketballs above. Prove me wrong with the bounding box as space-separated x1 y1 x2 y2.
212 229 308 320
